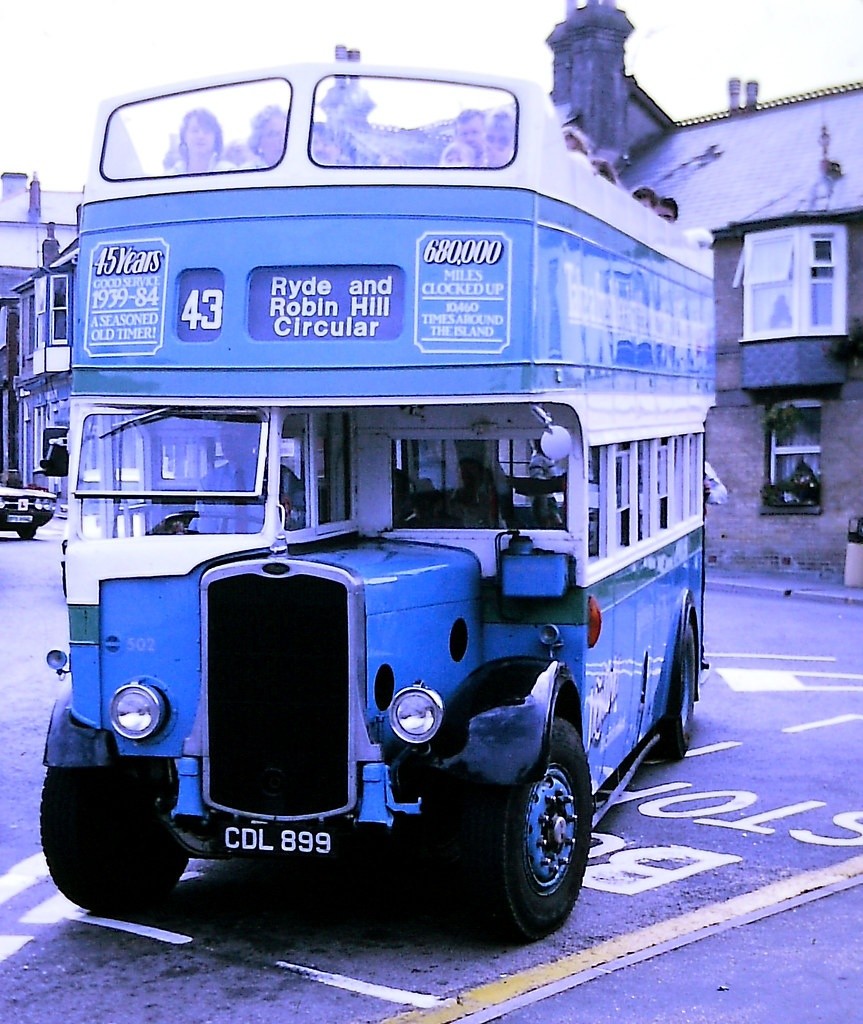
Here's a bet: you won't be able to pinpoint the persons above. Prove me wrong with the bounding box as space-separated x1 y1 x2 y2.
704 460 729 508
188 416 306 534
162 82 714 251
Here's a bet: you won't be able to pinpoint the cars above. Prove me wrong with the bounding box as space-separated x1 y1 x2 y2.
0 486 57 540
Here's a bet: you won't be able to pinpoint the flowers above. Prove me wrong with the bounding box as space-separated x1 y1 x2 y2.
776 466 818 500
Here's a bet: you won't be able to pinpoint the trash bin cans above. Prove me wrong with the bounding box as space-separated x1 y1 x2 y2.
844 516 863 588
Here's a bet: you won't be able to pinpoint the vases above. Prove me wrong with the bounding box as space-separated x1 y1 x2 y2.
784 490 800 504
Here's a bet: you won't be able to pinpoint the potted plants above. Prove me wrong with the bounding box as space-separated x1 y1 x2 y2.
762 405 803 439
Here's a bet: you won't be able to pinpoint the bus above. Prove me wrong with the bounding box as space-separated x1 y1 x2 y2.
35 60 724 947
